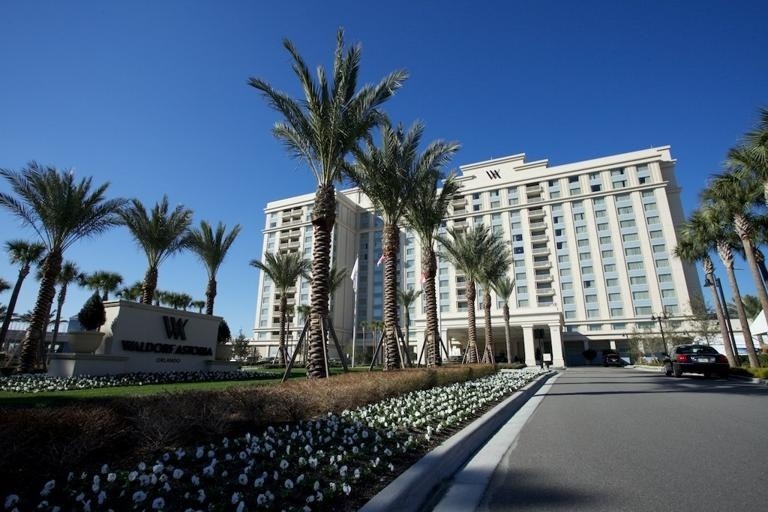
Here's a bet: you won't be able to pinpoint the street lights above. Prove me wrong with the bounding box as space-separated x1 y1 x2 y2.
649 311 669 355
702 271 741 367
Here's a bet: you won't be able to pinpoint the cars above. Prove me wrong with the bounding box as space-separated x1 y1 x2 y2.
662 344 730 380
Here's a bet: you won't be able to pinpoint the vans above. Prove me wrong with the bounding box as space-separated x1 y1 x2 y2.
604 353 619 367
